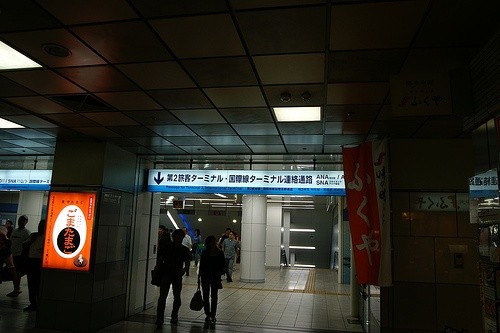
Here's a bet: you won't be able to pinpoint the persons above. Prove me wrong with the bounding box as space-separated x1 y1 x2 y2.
153 228 190 331
198 234 232 324
491 226 499 248
222 231 241 282
0 219 19 290
180 227 193 276
218 228 231 250
158 225 174 243
7 215 32 297
191 228 202 267
21 219 45 312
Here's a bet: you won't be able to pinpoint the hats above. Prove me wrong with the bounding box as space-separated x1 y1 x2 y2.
18 214 28 223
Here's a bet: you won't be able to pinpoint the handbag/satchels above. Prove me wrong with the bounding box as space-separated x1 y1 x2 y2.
151 262 167 286
190 286 204 311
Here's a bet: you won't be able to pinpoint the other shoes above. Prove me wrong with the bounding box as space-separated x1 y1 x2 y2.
210 313 216 322
205 317 211 321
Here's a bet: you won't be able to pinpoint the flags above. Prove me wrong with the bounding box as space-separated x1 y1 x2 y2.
341 135 393 288
